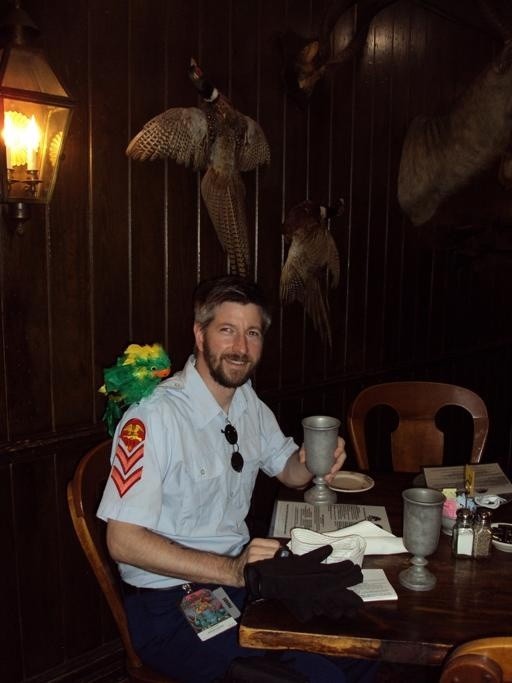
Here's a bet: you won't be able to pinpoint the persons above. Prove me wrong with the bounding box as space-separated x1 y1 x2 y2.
95 274 378 683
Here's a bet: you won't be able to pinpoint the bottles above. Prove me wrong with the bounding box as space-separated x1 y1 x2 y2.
450 508 474 559
473 510 493 558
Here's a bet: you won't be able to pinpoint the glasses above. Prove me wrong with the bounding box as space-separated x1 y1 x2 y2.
221 419 243 472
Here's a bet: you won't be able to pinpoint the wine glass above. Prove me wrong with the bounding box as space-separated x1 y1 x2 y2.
397 486 447 592
300 414 344 507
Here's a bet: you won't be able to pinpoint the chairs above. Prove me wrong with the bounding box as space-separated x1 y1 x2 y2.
66 438 176 683
439 636 512 683
347 381 490 473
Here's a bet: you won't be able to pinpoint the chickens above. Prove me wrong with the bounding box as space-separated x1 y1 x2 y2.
278 197 344 353
123 58 273 284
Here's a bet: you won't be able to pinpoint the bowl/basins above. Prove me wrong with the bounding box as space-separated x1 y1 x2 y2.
489 521 511 553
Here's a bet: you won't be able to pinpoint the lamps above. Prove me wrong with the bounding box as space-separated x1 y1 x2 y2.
0 9 75 237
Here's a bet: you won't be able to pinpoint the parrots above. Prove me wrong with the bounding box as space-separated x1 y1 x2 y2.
98 342 172 438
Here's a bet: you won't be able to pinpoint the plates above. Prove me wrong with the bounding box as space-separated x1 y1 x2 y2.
326 470 375 492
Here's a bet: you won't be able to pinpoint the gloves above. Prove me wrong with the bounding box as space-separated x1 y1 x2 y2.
242 545 365 629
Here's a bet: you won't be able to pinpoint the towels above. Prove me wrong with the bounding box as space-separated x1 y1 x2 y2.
288 520 409 555
